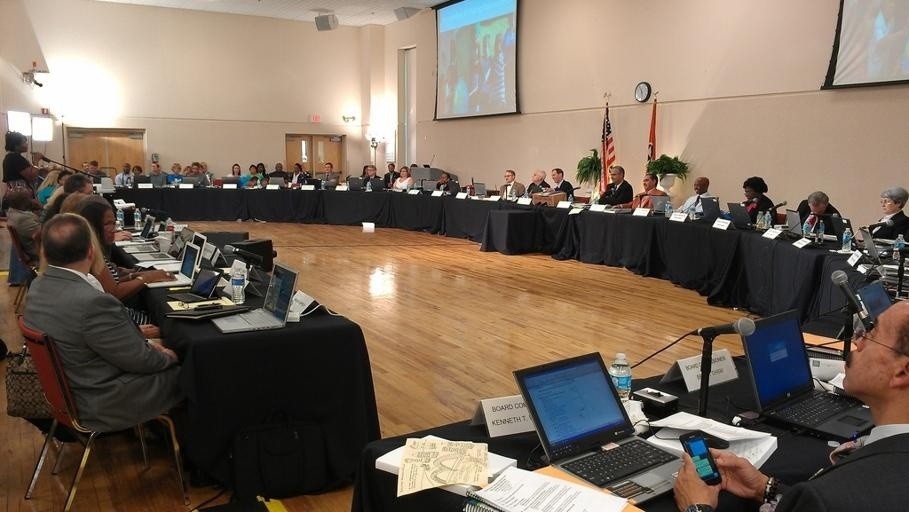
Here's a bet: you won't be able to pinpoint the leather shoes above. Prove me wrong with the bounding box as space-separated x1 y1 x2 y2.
190 466 219 487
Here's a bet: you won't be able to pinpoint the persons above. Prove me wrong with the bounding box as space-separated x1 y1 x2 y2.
446 33 506 115
868 1 903 72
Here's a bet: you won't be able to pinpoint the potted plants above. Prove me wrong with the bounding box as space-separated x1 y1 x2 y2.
646 155 689 195
576 149 603 193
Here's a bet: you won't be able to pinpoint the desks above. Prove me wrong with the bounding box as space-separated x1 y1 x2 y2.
109 229 382 507
737 229 880 338
397 188 577 261
576 209 738 308
102 191 396 226
345 357 873 512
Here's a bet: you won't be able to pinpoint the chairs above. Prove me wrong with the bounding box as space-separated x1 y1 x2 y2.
91 414 189 506
15 309 191 510
6 224 40 312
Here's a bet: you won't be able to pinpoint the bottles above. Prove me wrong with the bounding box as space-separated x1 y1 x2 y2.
133 209 142 230
814 219 825 245
321 182 325 189
568 194 573 203
756 209 771 228
365 181 371 191
610 353 631 401
663 200 673 218
893 235 905 261
117 209 124 227
802 220 810 239
230 268 246 303
843 228 852 252
689 204 695 221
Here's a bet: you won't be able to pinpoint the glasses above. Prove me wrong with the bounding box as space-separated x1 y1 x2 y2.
856 328 909 359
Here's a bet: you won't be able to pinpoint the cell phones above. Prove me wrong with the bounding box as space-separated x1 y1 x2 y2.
679 430 722 485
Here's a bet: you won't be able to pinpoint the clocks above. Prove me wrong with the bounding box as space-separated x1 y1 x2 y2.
633 81 652 103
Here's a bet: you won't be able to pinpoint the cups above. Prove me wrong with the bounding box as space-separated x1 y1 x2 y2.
158 231 172 252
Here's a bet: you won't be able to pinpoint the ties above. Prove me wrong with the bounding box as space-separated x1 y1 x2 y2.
805 215 816 233
695 196 700 207
829 435 870 465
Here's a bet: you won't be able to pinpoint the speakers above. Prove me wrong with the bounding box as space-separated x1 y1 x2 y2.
315 14 339 32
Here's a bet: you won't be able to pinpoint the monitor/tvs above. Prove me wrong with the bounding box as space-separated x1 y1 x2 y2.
424 165 430 168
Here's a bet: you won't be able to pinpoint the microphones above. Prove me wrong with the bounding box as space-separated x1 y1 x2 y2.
30 151 50 164
429 155 435 165
869 220 894 227
831 270 874 330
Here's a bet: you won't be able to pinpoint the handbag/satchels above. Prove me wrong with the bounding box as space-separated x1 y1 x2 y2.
5 352 53 419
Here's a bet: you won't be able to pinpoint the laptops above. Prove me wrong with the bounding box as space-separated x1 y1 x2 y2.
166 264 225 303
151 175 166 188
815 214 866 249
162 232 207 273
857 279 892 323
422 180 441 193
114 215 196 261
221 176 241 189
136 240 200 289
240 175 252 187
741 309 875 442
183 176 201 187
306 178 321 189
133 175 152 188
786 209 804 239
653 196 673 214
859 227 909 271
213 177 223 186
473 183 485 196
268 177 285 188
513 352 683 505
701 197 725 221
210 262 299 334
370 180 384 192
727 202 757 231
349 177 362 191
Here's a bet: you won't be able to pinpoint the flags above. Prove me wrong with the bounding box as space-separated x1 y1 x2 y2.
599 108 616 197
648 103 656 167
